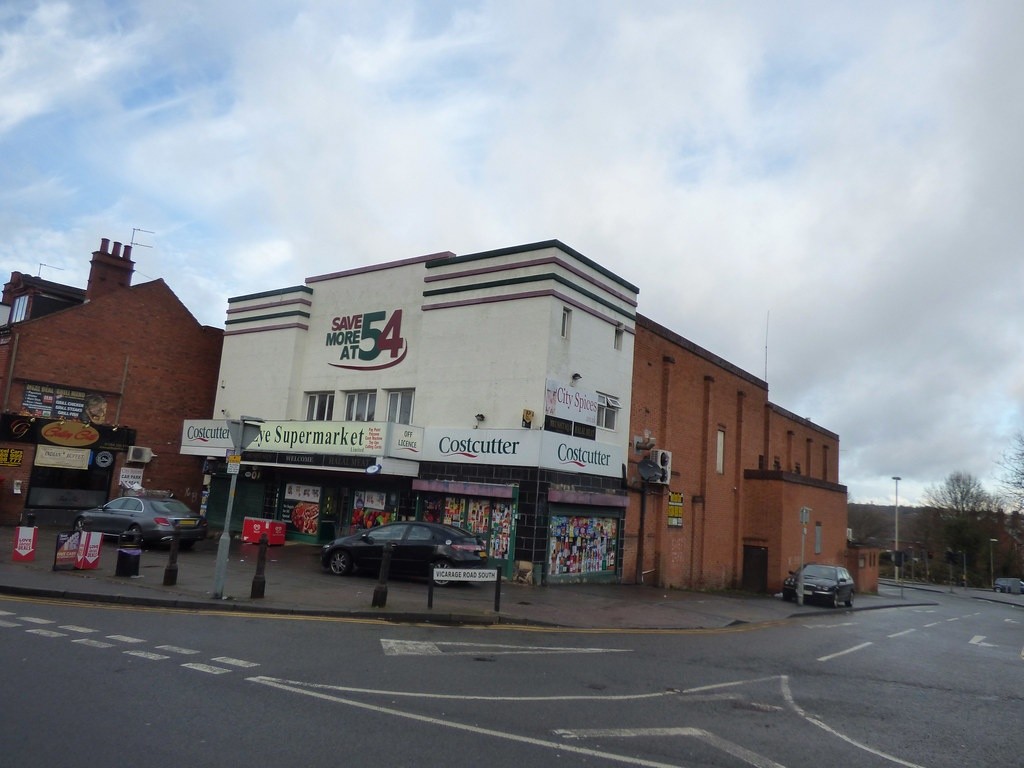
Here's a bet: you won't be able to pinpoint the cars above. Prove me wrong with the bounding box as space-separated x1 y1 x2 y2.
320 521 488 587
993 578 1024 593
71 496 208 550
784 562 856 607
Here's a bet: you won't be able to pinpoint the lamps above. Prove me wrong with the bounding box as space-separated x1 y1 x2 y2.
572 373 583 380
30 416 35 422
112 426 119 431
59 418 65 425
476 414 485 422
85 422 91 429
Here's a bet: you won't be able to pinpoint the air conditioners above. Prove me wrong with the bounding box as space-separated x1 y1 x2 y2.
127 446 151 463
649 448 672 484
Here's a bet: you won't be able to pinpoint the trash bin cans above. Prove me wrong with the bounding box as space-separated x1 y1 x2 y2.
115 531 142 578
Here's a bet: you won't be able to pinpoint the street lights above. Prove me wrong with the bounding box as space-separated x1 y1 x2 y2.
892 476 901 583
989 538 997 587
